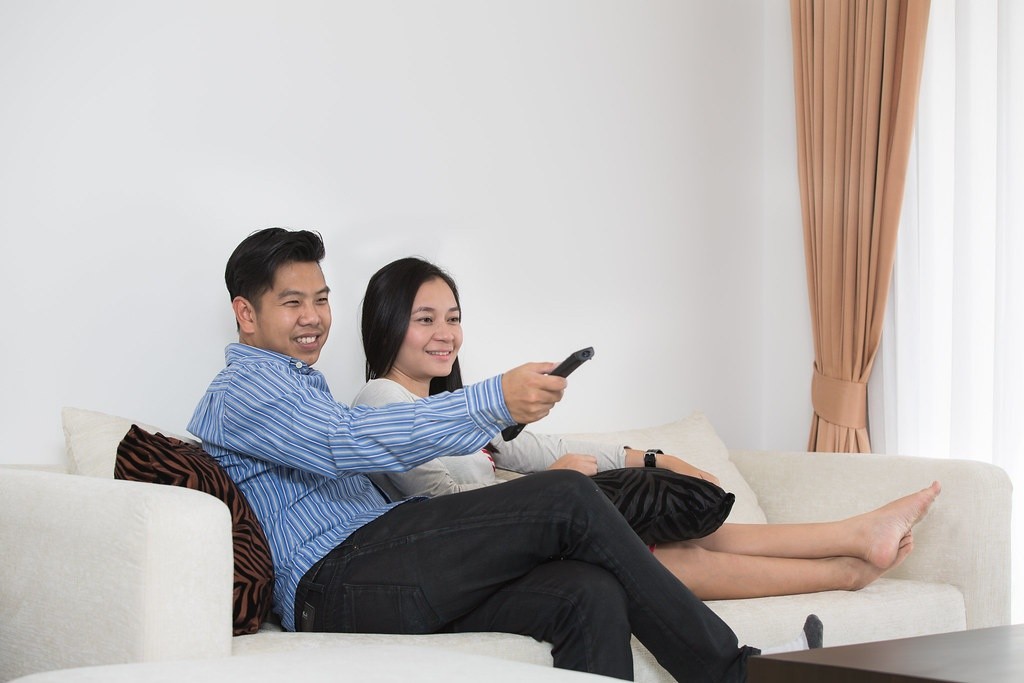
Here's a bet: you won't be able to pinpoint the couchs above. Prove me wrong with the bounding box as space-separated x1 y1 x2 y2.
0 449 1014 683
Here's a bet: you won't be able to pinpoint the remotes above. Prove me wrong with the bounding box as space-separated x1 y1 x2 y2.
501 346 594 441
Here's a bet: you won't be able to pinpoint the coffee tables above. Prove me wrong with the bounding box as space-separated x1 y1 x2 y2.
741 624 1024 683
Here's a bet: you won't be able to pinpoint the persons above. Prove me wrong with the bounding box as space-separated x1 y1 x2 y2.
185 228 827 683
351 257 939 602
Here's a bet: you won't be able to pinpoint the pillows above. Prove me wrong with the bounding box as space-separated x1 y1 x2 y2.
114 422 272 638
489 411 775 523
59 406 207 475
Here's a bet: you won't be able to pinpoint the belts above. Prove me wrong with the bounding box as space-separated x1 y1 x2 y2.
295 555 329 632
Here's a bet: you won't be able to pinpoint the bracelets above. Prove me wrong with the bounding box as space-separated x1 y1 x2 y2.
643 448 664 467
